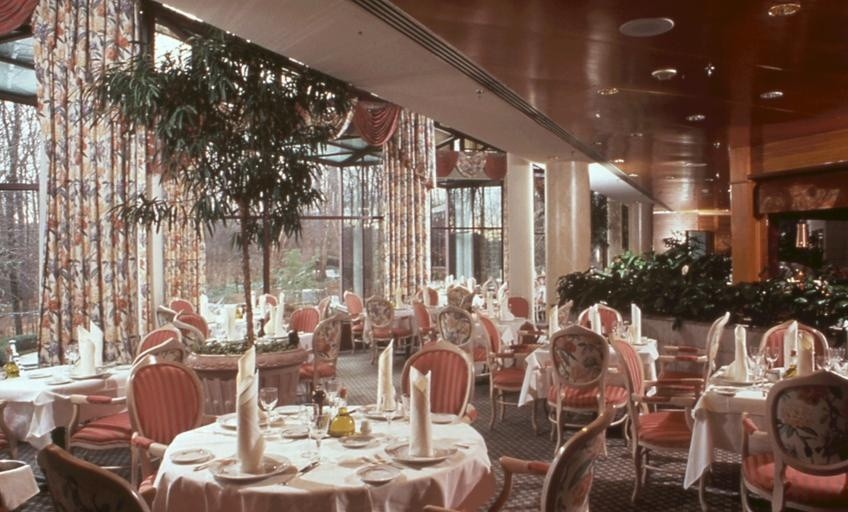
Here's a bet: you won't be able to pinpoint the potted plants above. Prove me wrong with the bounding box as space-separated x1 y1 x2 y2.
67 32 361 412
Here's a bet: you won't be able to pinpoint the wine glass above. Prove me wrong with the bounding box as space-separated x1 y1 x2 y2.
297 403 331 465
742 343 845 383
610 319 633 342
62 344 79 379
377 384 398 444
257 388 280 434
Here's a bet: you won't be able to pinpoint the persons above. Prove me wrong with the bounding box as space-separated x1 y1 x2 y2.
808 235 820 261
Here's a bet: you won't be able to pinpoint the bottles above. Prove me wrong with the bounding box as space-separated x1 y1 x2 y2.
0 338 23 379
311 382 356 438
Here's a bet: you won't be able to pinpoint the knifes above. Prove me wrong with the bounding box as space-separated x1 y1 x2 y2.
281 461 321 487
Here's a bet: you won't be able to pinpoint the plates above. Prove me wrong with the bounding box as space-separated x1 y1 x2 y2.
209 455 290 482
429 412 458 425
385 444 459 465
282 426 310 438
338 435 375 449
258 405 307 426
219 411 238 429
354 465 401 486
359 403 401 421
168 447 210 466
